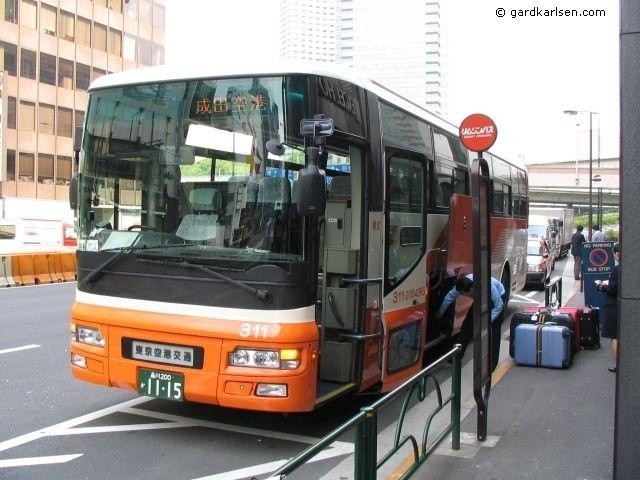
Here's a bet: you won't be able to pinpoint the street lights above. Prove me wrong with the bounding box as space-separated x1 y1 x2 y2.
564 108 602 242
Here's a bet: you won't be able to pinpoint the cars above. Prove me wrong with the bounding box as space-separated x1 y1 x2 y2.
525 238 555 291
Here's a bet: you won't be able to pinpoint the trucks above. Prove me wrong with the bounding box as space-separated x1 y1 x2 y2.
528 207 574 271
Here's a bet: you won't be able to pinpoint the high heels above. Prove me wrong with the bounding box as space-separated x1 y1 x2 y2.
608 365 617 372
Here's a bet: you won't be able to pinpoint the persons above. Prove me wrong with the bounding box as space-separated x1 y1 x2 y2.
571 225 585 281
592 225 605 242
434 273 506 373
596 243 620 372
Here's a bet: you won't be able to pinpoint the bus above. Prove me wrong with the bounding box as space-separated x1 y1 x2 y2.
70 54 528 414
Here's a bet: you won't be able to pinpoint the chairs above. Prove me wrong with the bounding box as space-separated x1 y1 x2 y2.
174 174 466 246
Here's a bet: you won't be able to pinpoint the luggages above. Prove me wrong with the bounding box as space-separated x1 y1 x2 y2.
509 304 601 370
